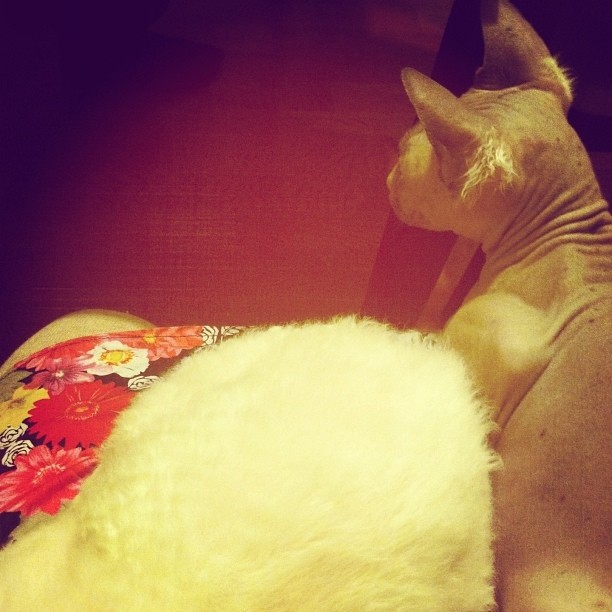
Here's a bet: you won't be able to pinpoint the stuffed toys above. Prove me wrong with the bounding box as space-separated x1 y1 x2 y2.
2 315 503 611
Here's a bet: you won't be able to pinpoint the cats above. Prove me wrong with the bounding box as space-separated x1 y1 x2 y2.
384 1 611 611
